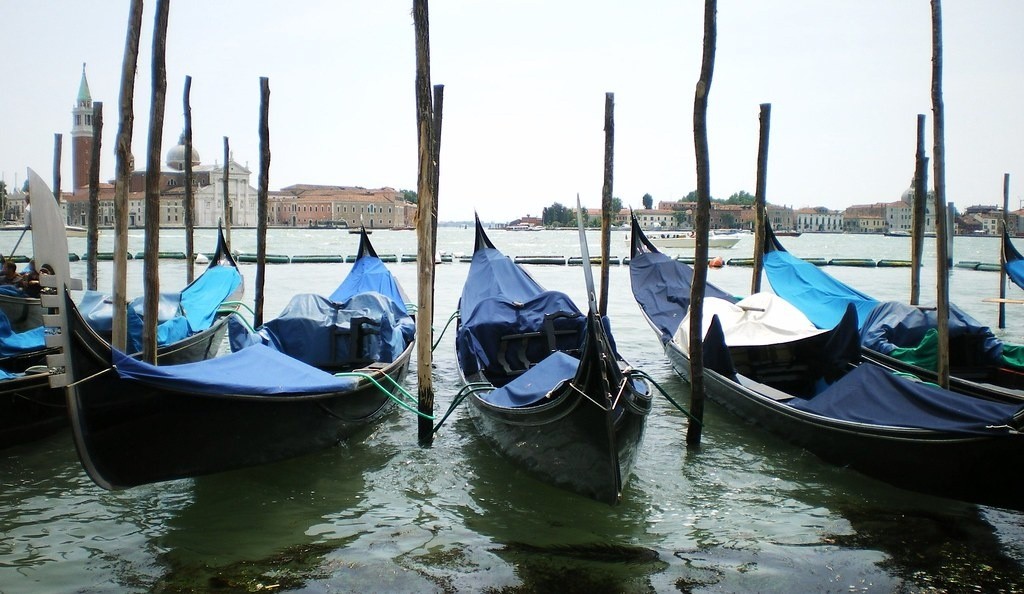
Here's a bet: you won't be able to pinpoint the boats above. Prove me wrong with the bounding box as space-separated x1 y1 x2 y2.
23 165 418 495
0 253 81 339
390 224 417 231
741 203 1023 427
454 209 652 507
623 206 1023 453
506 222 546 231
882 231 938 237
773 230 801 237
0 212 244 453
710 229 751 236
620 235 743 252
348 229 372 236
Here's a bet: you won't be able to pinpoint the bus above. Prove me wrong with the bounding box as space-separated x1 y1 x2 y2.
313 219 350 229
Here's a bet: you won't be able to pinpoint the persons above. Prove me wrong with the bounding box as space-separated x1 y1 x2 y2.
0 261 23 288
22 260 44 294
